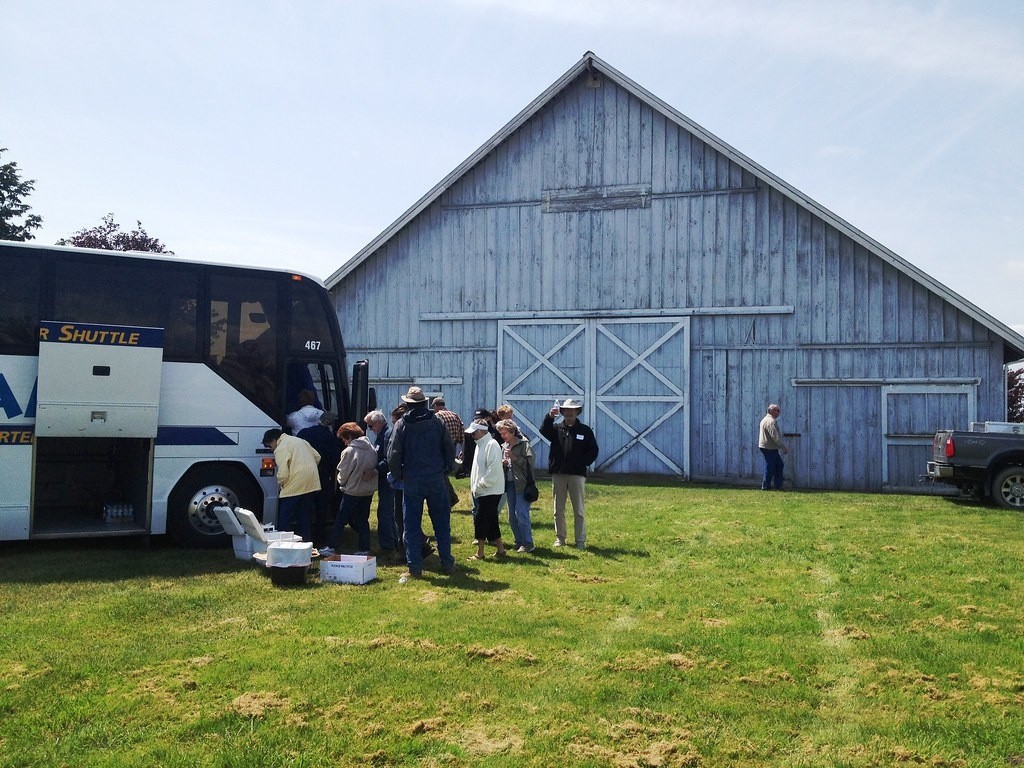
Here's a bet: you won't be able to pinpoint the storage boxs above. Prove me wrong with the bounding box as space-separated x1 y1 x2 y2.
213 507 377 587
970 421 1024 435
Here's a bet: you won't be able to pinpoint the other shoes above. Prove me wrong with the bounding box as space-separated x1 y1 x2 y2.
518 542 535 553
510 543 518 550
472 539 478 544
467 554 485 561
576 541 585 549
554 536 565 546
422 540 436 559
492 549 506 556
400 572 416 578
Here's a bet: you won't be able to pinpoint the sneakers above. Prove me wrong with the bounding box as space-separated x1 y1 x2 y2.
319 546 336 557
352 551 370 556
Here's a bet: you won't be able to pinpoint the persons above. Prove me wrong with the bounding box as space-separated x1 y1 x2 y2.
539 398 599 550
261 385 539 582
758 404 788 491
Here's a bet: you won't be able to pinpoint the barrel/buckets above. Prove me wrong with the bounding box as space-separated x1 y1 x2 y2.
307 552 320 573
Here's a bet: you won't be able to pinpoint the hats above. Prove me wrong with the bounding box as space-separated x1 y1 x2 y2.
559 399 582 417
320 411 335 425
473 409 488 419
401 387 429 403
464 419 489 433
431 397 445 406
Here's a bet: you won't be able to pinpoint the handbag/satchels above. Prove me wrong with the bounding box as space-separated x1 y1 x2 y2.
522 457 538 503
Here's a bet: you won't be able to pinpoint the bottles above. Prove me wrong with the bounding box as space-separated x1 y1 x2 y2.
553 399 560 416
504 450 511 464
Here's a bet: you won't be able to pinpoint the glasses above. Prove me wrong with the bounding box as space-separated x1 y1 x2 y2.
774 410 779 411
368 423 374 428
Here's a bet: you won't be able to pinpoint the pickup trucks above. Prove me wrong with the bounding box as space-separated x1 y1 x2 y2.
927 428 1023 512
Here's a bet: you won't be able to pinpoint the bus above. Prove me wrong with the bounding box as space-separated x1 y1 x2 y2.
0 239 379 551
